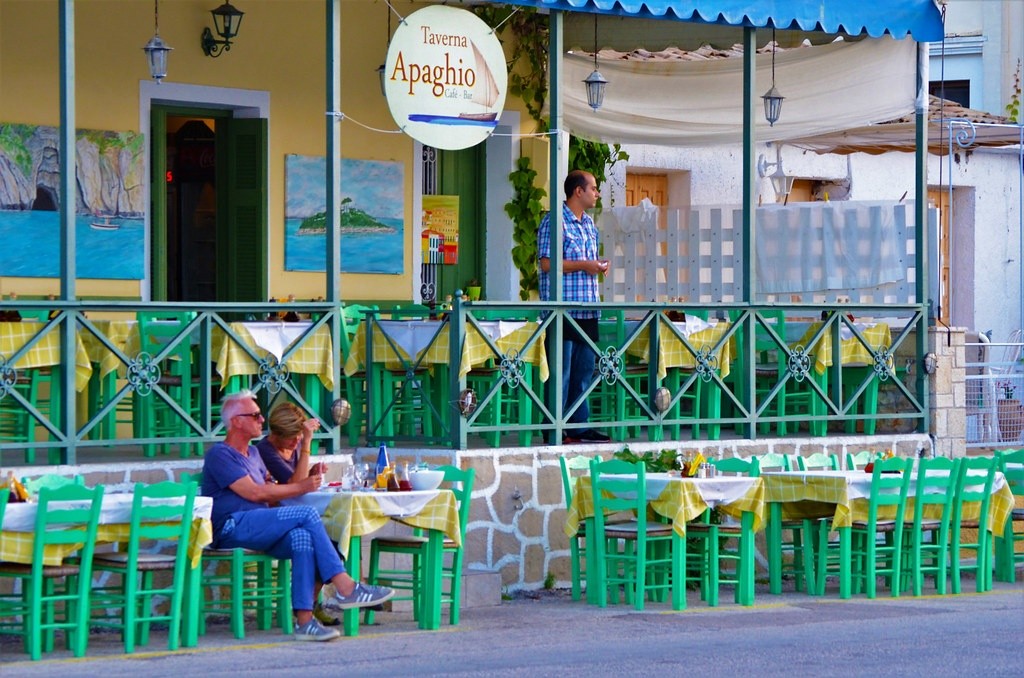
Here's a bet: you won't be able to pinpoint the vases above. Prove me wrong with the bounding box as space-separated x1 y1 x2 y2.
983 399 1024 441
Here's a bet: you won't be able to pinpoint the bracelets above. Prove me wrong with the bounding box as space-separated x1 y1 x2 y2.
301 450 309 453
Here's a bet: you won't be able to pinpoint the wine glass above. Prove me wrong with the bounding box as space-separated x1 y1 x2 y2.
355 463 369 492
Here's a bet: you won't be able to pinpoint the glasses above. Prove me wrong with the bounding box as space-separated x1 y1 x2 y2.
237 410 263 420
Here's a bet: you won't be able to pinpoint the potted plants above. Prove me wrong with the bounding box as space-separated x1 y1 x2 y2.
466 279 481 300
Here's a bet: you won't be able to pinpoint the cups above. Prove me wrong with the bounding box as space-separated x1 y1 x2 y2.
375 466 388 491
341 466 355 491
467 286 481 300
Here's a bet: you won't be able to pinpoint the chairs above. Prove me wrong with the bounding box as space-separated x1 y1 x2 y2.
0 307 1024 662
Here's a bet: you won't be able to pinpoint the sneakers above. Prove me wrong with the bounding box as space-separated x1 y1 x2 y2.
331 581 396 607
542 426 581 444
316 605 340 626
294 618 341 640
574 429 610 443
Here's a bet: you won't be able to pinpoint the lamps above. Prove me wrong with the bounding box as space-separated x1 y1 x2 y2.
761 28 786 127
200 0 245 58
141 0 174 85
376 0 394 98
580 14 610 112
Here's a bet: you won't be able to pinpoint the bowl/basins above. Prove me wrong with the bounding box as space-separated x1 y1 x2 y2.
407 470 445 490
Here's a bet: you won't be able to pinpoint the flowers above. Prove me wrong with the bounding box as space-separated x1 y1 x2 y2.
994 380 1016 399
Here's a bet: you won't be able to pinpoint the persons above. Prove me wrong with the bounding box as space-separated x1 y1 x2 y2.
537 169 611 444
201 389 396 640
256 402 345 626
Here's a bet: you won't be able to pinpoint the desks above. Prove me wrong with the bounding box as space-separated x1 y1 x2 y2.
761 469 1015 599
598 321 736 441
76 319 216 458
564 472 766 612
0 320 93 465
756 321 895 437
343 320 549 449
210 321 334 456
276 489 462 636
0 493 213 647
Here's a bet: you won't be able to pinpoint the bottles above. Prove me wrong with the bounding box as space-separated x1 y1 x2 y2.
442 296 452 322
399 460 412 490
864 448 876 473
680 450 716 479
283 295 301 322
821 298 854 322
388 461 400 491
6 471 19 502
373 442 389 481
668 297 687 321
